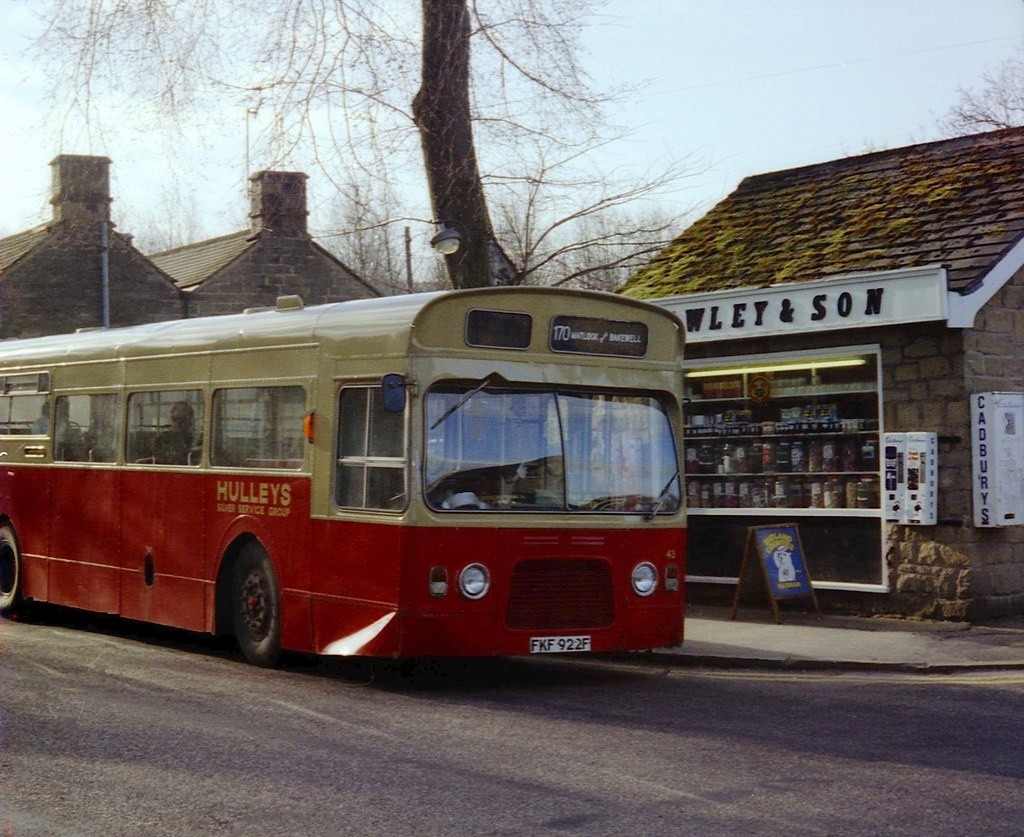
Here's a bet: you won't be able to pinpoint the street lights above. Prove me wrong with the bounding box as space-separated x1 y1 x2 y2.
427 216 522 286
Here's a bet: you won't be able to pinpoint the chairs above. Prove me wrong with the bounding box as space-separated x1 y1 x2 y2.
0 419 305 469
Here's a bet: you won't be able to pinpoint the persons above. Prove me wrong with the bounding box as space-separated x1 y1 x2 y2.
32 398 88 460
443 400 528 495
150 402 201 466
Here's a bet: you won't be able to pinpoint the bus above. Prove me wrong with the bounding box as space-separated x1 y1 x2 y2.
1 285 691 681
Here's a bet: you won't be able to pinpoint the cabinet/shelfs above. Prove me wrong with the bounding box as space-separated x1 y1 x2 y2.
683 369 879 476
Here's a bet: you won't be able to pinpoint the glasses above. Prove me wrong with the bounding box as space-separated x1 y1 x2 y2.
171 415 192 422
